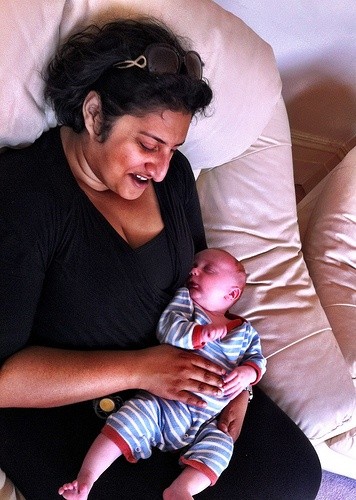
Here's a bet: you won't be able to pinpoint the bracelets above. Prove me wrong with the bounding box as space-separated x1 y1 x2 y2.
243 385 254 404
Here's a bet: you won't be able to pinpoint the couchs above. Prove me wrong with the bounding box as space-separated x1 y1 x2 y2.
0 0 356 500
296 146 356 482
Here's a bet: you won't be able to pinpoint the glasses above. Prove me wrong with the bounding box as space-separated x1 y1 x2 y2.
115 44 205 80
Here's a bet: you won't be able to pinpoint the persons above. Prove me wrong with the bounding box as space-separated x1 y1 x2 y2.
57 247 267 500
0 20 322 500
